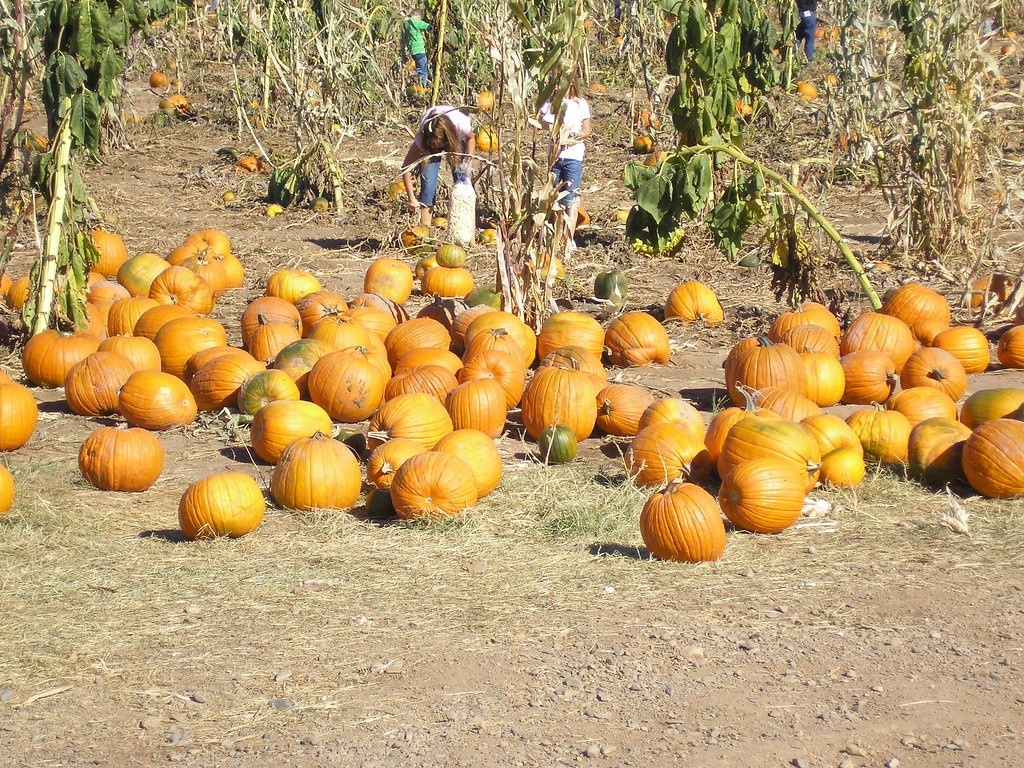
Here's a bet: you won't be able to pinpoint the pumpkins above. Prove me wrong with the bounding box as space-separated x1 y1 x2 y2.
0 30 1024 562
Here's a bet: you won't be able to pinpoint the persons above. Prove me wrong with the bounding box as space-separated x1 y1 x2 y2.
404 9 430 86
540 72 590 249
613 0 641 37
402 106 475 225
796 0 822 64
